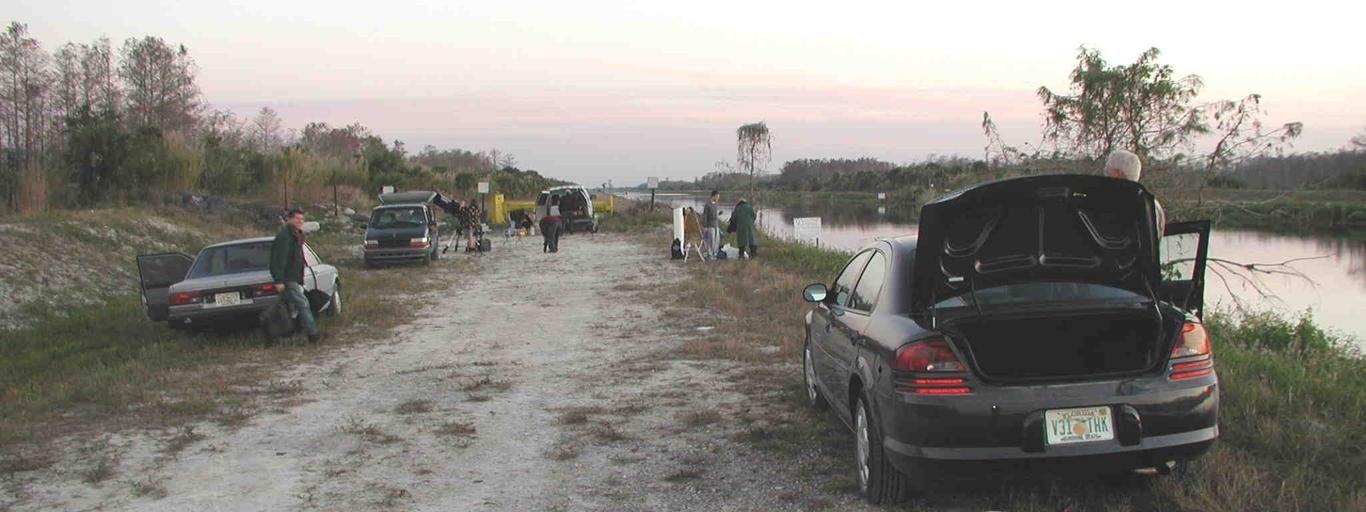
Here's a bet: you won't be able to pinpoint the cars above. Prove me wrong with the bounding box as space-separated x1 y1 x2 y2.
363 188 442 265
133 235 344 339
797 172 1225 506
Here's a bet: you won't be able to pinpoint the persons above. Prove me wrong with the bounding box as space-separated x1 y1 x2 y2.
729 201 757 261
256 210 329 344
559 190 577 235
701 191 720 262
540 216 562 252
466 199 480 251
1104 150 1166 243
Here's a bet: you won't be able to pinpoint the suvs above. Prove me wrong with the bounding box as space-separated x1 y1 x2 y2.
532 184 599 237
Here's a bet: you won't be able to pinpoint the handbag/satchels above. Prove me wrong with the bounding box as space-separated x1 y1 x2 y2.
304 288 329 314
259 291 300 339
